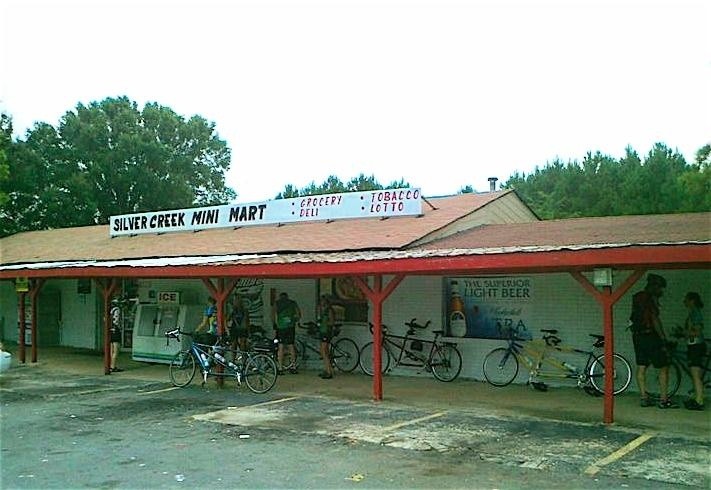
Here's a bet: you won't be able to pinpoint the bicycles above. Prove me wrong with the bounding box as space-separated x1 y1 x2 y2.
359 317 462 382
637 326 710 401
482 319 632 397
163 318 359 394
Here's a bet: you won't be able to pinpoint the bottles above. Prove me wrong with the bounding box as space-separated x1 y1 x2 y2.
448 280 467 338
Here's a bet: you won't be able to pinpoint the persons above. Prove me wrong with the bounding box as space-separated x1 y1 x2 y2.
316 293 337 380
673 290 708 411
108 296 125 372
191 296 220 369
633 272 682 409
271 292 302 376
228 297 251 371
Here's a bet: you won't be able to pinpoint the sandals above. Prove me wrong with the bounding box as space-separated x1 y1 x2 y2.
110 367 124 372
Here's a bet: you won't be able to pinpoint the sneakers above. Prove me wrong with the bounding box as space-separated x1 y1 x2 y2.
278 368 298 375
640 397 705 411
317 370 332 379
